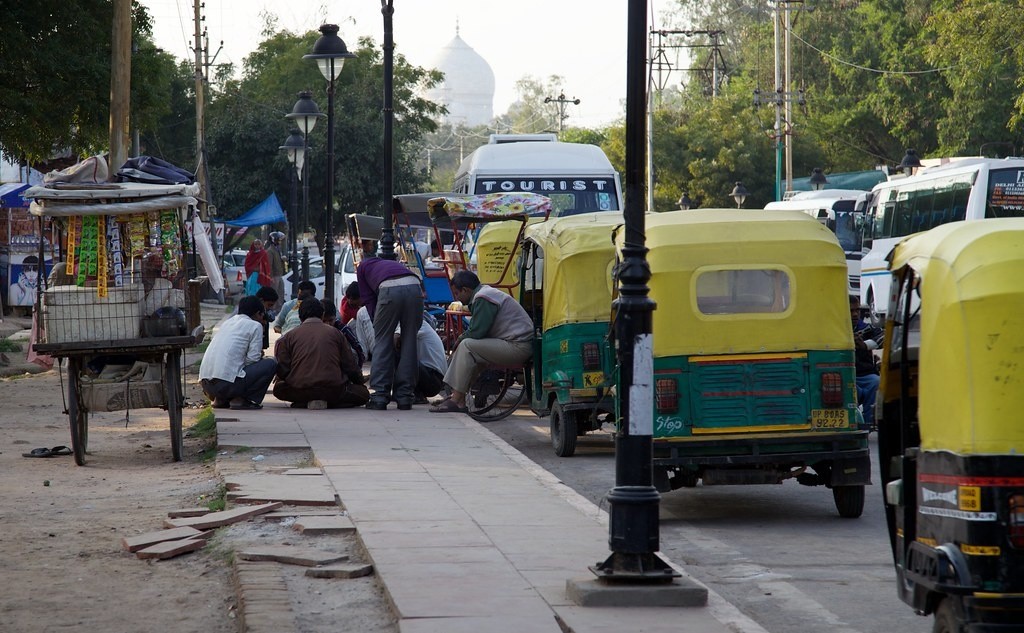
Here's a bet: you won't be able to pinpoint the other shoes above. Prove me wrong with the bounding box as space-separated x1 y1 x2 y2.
397 402 412 410
365 399 387 410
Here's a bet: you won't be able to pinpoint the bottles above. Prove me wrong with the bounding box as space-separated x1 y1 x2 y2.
18 272 44 289
54 242 59 257
11 235 50 252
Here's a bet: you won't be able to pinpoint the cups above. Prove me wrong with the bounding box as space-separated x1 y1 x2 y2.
192 217 225 293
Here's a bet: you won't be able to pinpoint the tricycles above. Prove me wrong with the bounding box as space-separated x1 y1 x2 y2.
24 183 206 467
346 192 552 422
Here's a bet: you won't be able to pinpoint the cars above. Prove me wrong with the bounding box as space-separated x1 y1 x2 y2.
185 249 250 297
281 238 401 318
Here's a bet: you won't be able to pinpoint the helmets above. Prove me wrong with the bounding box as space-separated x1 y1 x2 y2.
268 231 286 246
148 305 187 336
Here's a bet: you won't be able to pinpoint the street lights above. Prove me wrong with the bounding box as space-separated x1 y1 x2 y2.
730 184 751 209
301 25 358 304
281 93 328 257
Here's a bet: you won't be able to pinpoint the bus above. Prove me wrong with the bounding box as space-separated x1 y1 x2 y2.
454 134 624 290
854 156 1024 326
764 188 869 312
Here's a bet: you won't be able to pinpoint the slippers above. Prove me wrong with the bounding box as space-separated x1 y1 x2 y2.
429 395 466 412
230 400 263 409
412 395 429 404
211 400 230 408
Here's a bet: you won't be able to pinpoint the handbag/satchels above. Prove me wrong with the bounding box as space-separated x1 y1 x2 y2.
25 288 54 369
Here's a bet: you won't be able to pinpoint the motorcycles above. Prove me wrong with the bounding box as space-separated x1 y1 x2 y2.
611 210 872 519
875 216 1024 633
521 210 658 458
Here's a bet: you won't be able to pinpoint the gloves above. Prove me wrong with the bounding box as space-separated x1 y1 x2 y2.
863 339 879 350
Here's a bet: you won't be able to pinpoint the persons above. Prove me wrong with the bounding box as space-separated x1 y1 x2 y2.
198 255 534 413
848 293 881 432
409 240 444 265
266 231 285 316
10 256 43 306
134 252 173 317
244 238 270 297
